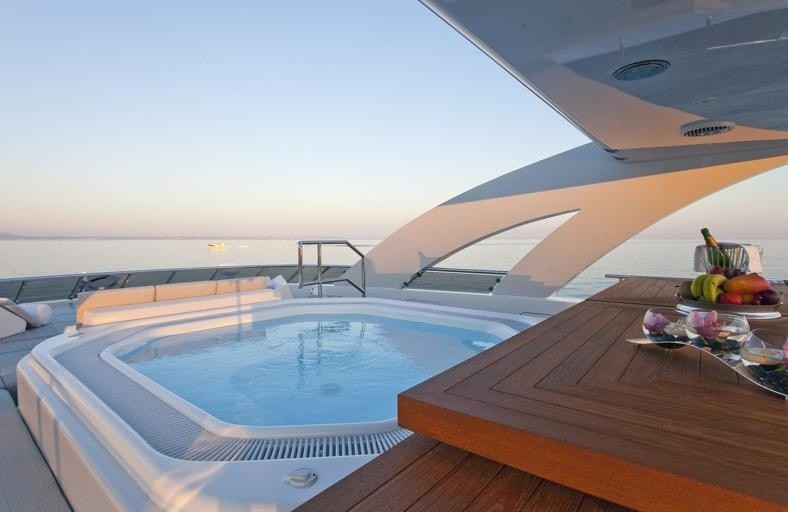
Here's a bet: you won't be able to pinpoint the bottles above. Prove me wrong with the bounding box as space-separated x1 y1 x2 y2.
701 227 732 270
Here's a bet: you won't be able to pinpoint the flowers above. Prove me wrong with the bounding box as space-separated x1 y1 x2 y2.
750 335 788 392
687 305 729 349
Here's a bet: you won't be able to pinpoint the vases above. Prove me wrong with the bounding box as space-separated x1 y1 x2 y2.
687 305 750 352
739 322 788 393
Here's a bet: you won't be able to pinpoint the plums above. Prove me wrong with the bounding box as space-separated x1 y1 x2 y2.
752 295 763 305
761 289 779 305
710 267 720 274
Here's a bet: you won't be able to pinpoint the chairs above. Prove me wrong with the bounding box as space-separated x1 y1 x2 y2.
742 245 766 276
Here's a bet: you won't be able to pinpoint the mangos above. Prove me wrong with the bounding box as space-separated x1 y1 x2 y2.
721 274 770 293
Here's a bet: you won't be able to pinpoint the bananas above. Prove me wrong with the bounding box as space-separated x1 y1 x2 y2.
690 274 725 303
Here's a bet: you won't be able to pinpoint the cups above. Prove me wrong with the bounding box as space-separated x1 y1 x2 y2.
642 305 788 381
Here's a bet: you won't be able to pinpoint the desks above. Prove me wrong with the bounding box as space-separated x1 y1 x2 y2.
387 272 788 510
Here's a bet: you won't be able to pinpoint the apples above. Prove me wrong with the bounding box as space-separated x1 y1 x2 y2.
719 292 742 305
680 279 698 299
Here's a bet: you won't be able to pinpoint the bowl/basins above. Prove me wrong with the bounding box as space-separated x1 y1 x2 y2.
699 246 747 274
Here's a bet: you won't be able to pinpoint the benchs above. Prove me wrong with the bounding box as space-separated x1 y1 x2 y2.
70 272 283 327
